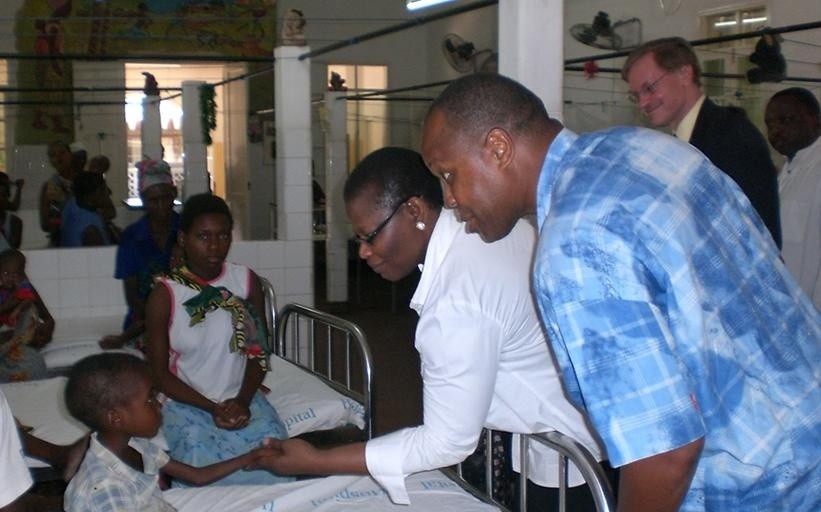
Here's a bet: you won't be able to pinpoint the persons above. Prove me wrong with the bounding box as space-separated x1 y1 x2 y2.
621 37 781 254
765 87 820 312
420 73 821 512
1 139 285 512
245 145 618 512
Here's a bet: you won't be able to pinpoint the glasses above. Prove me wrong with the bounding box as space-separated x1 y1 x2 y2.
346 190 423 246
623 62 680 103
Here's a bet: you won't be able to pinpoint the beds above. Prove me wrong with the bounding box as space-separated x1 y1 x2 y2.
1 275 274 375
164 421 613 512
0 304 370 489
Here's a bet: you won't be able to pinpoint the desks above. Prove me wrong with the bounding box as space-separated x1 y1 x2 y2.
274 202 328 243
121 197 182 210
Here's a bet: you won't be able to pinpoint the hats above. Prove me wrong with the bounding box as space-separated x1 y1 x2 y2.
135 159 173 195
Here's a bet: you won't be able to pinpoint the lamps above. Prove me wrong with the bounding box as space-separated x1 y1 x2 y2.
747 36 786 85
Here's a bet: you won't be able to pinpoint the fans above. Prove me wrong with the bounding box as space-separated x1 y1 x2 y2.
440 33 496 73
568 11 644 50
